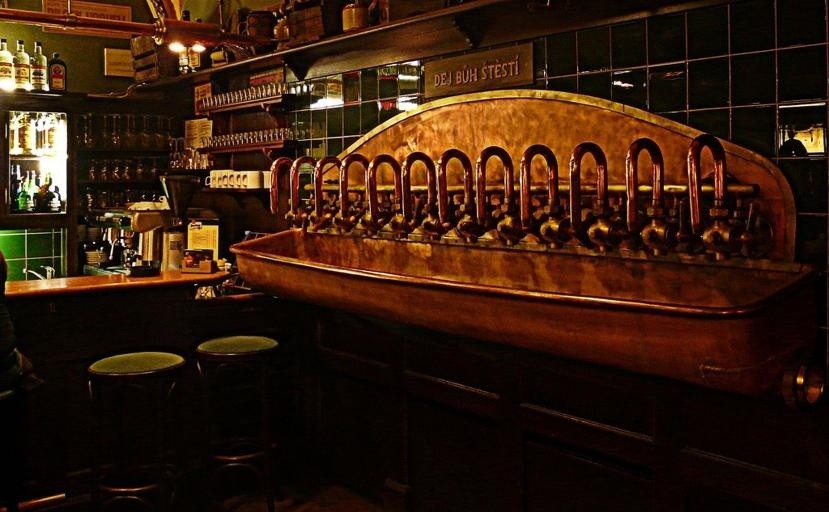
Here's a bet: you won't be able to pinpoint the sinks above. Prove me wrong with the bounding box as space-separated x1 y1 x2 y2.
228 226 820 395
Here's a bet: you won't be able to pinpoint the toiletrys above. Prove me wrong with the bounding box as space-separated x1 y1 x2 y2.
18 181 32 210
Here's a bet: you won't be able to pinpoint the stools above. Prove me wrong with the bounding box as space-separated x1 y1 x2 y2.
87 351 186 511
196 335 279 510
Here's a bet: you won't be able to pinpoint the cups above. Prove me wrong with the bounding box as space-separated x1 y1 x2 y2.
200 128 293 149
198 82 287 110
205 169 272 189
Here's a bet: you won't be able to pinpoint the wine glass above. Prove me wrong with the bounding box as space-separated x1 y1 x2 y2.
78 111 177 211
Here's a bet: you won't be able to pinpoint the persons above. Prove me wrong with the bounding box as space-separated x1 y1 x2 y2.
0 252 45 395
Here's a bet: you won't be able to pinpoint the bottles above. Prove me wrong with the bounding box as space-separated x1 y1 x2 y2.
0 36 15 93
30 42 50 93
13 40 30 93
78 227 111 268
48 53 68 94
178 39 208 76
7 111 68 215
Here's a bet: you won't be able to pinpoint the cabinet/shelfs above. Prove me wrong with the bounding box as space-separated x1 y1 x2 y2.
178 50 295 221
0 88 179 221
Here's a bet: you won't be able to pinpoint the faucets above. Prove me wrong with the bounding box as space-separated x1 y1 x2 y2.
269 134 769 261
22 264 56 280
